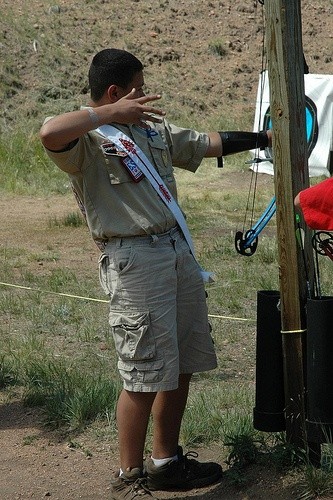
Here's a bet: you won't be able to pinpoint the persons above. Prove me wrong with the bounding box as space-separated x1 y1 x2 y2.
290 174 332 231
37 46 274 500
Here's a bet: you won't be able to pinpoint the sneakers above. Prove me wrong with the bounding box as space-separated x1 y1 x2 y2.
111 467 159 499
146 446 223 490
